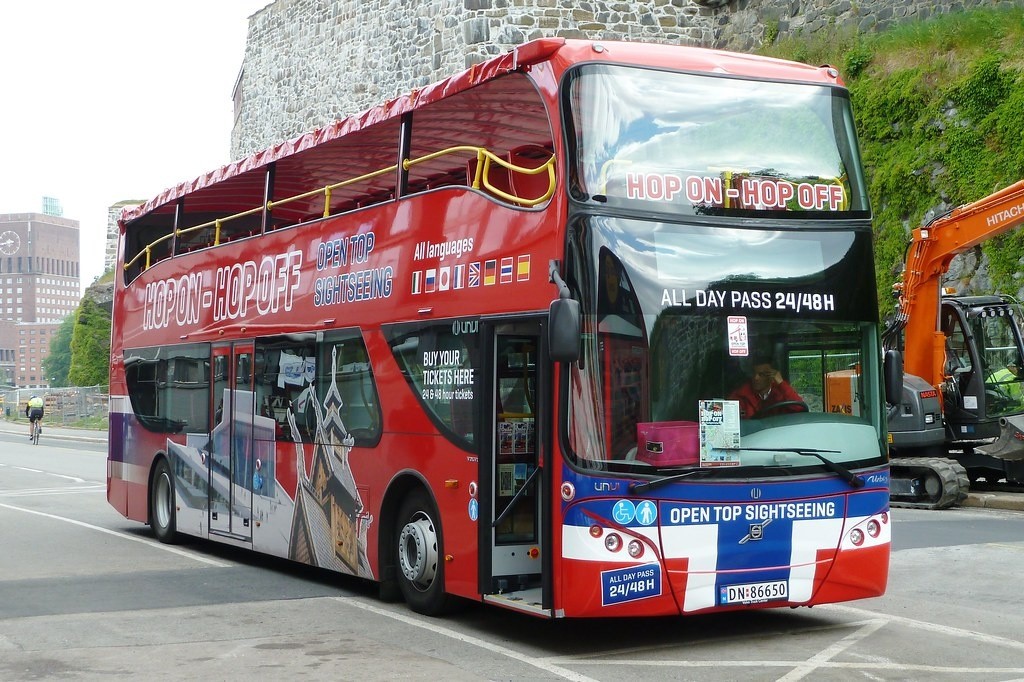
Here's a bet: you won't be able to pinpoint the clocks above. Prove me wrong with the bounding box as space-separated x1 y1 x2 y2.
0 231 21 255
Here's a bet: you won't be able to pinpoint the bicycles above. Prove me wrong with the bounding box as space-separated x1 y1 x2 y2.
26 415 41 445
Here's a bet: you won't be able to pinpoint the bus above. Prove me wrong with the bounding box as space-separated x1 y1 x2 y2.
106 37 905 620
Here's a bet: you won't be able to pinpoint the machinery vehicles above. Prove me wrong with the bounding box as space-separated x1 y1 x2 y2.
820 180 1024 511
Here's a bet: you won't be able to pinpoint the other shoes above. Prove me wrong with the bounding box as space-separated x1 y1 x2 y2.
39 429 42 434
30 435 33 440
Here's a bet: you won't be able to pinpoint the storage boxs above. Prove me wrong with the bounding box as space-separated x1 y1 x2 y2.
635 421 700 467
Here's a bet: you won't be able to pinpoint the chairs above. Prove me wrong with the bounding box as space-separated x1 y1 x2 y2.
140 144 556 274
944 340 960 375
605 173 786 210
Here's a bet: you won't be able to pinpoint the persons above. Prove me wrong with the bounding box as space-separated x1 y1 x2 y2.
214 399 223 429
985 362 1024 415
731 355 807 421
607 372 683 460
273 417 294 443
366 391 379 431
504 377 531 414
26 395 44 440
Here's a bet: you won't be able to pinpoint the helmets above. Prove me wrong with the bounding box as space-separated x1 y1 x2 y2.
30 396 37 399
